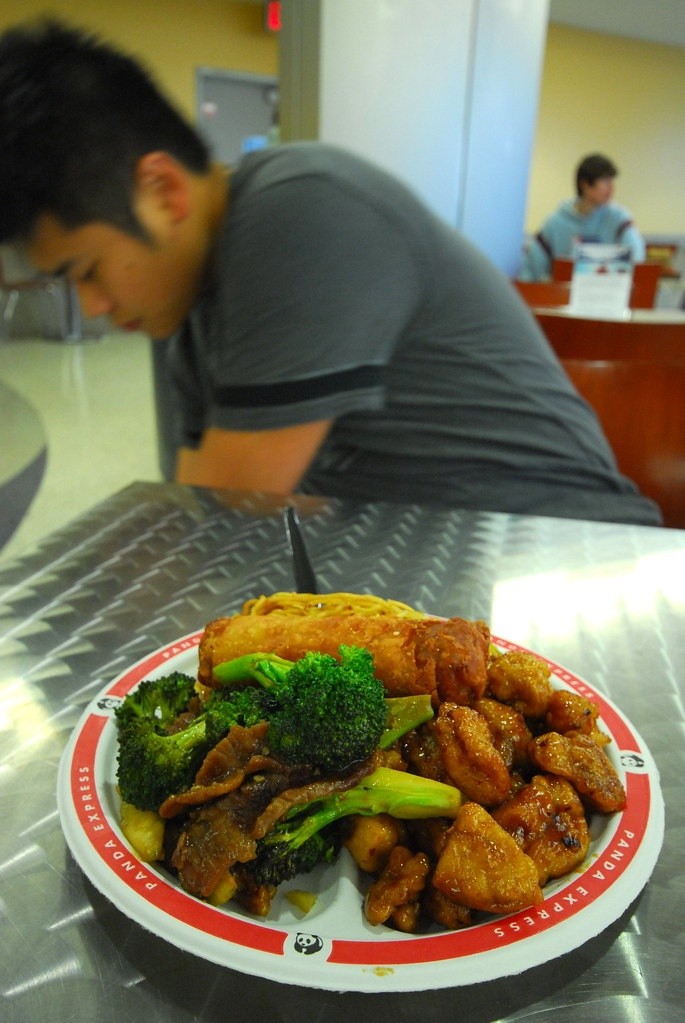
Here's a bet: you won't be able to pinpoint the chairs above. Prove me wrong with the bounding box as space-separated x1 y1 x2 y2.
554 356 685 529
0 379 46 544
511 237 684 361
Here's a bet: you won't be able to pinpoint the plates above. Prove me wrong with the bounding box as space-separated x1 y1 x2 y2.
57 628 665 994
574 243 627 260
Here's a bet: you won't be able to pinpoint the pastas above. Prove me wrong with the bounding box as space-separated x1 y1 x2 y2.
240 592 434 620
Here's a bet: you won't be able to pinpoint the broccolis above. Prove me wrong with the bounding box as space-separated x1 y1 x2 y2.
110 644 462 888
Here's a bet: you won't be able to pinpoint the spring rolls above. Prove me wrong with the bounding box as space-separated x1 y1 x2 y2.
195 614 491 707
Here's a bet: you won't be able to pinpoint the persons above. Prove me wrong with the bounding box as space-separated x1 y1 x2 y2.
0 9 668 525
517 153 647 281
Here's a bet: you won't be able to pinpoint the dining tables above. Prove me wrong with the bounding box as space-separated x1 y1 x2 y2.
0 482 685 1023
